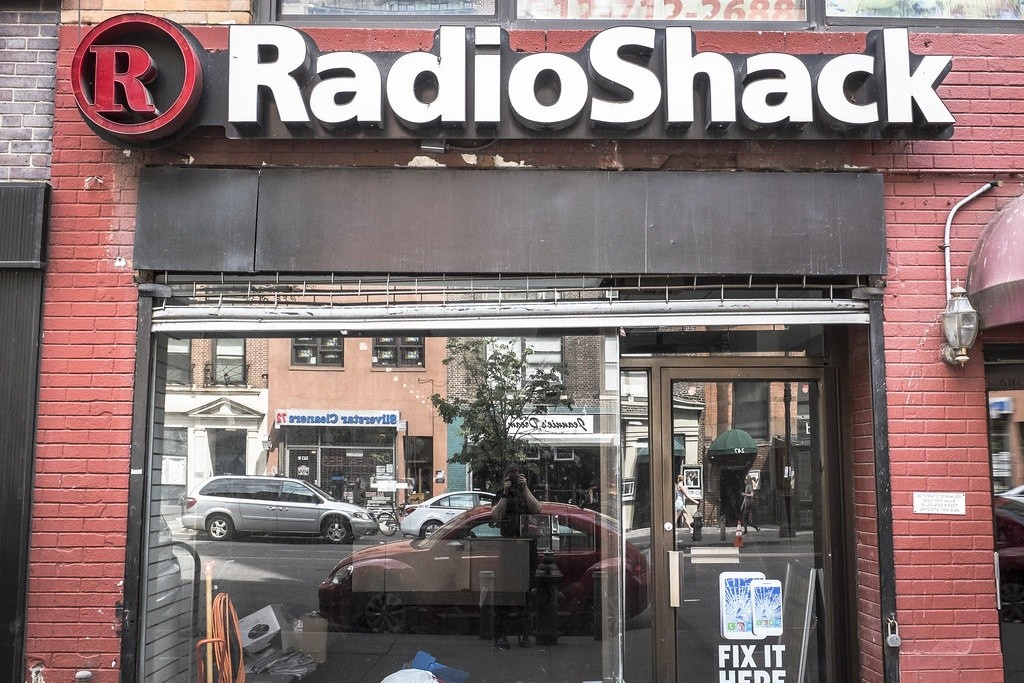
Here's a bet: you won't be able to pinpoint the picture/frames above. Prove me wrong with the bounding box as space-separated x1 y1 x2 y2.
748 470 761 490
683 468 701 489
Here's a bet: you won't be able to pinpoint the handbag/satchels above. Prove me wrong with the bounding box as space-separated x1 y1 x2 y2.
744 485 754 508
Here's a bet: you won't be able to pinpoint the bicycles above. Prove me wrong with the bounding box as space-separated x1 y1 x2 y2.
378 499 408 537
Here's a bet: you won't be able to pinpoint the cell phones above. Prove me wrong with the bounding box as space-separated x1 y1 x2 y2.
505 476 521 483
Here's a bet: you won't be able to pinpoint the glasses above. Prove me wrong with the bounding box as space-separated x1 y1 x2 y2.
745 479 748 480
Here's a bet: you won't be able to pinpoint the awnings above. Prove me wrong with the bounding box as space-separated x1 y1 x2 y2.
963 190 1024 324
707 428 759 456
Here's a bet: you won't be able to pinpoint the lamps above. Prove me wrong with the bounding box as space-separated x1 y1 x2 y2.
941 277 979 367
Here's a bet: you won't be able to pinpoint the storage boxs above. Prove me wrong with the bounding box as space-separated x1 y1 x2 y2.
281 618 328 663
237 604 281 657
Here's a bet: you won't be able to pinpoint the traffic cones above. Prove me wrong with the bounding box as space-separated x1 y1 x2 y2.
733 520 745 548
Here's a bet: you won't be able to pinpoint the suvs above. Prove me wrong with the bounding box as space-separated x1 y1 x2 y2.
181 473 379 545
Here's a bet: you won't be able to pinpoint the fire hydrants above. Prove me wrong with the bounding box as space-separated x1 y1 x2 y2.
529 550 567 647
690 510 704 542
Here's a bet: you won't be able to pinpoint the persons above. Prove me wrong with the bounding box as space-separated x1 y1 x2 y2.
740 475 762 535
351 477 365 509
675 475 699 534
491 463 543 649
686 471 698 486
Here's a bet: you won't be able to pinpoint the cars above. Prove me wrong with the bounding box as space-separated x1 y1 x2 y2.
318 501 653 634
993 484 1024 625
400 487 572 539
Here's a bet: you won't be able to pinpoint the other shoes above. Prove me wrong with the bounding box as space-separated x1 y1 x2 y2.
690 527 693 534
757 528 761 534
496 634 511 651
742 531 747 536
518 635 532 648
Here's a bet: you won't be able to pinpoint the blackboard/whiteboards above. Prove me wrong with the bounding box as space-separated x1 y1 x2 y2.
778 559 817 683
